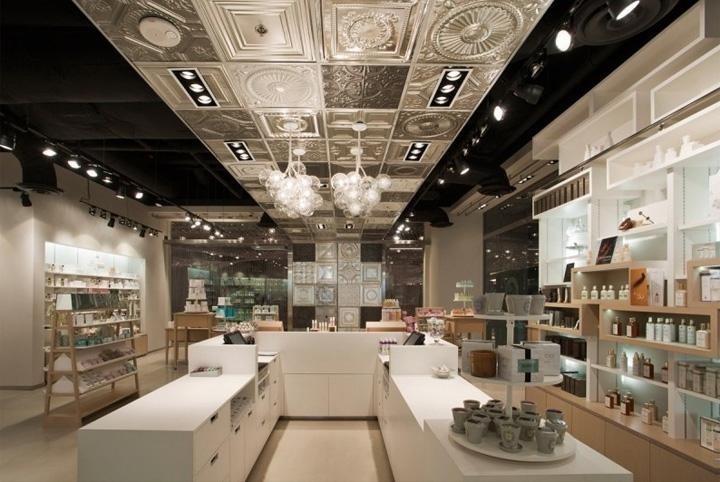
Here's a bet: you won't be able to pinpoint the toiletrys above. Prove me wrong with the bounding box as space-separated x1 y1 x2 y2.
662 361 668 383
627 317 637 337
625 284 630 300
620 352 627 372
663 319 672 342
670 319 676 340
697 323 709 348
628 393 635 411
633 353 640 376
650 400 657 421
646 317 655 340
581 286 587 300
613 389 621 406
606 349 615 368
655 318 662 341
600 284 607 300
605 390 615 408
640 353 645 375
591 286 597 300
619 285 625 299
640 402 651 425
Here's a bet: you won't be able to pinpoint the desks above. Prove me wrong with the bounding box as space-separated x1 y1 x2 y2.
444 315 486 352
365 321 407 331
253 320 283 331
165 326 211 366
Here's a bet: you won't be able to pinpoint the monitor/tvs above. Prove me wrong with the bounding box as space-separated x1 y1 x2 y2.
401 331 425 345
223 330 247 344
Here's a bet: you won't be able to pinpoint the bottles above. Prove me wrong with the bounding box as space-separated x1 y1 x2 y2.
610 388 620 405
649 400 658 421
580 286 588 299
645 316 654 341
621 352 627 371
625 317 636 338
641 402 653 425
590 285 598 300
632 352 641 376
626 392 635 415
600 285 608 300
604 389 614 408
675 283 687 307
624 285 630 299
669 319 676 342
618 284 625 300
654 317 663 342
605 348 615 368
662 318 671 343
696 323 710 348
639 353 645 377
660 361 668 383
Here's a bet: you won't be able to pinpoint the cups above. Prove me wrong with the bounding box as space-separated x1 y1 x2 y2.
452 399 567 453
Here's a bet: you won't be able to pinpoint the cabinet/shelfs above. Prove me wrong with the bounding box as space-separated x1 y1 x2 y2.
43 242 148 386
270 353 280 435
173 312 214 370
257 364 270 460
231 375 257 482
526 1 719 481
192 399 230 482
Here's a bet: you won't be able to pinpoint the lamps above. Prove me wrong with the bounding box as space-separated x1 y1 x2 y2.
606 0 641 21
258 122 323 220
331 123 392 217
